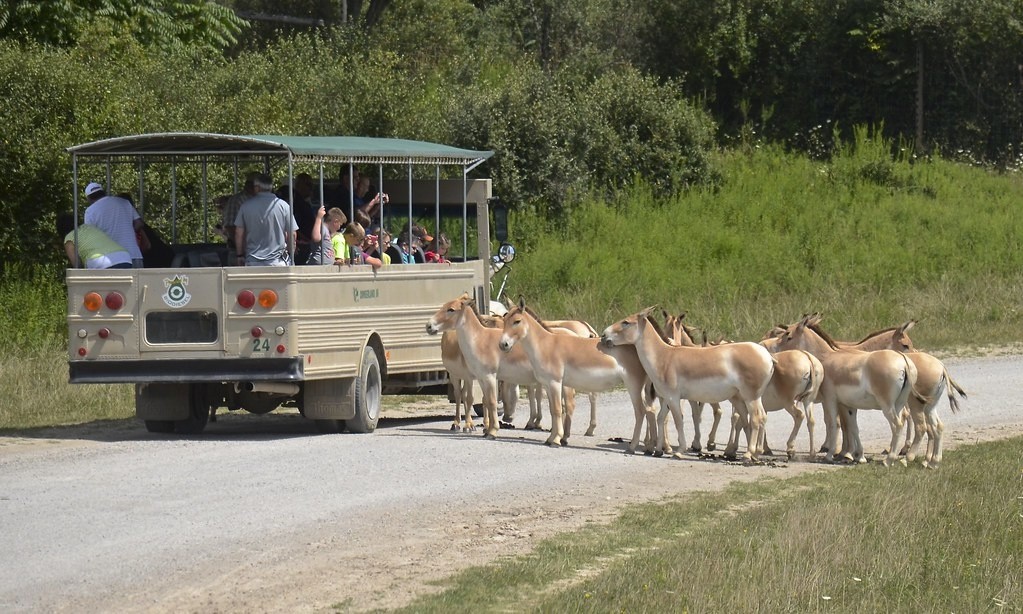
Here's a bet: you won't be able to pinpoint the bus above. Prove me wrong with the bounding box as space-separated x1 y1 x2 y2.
64 130 516 434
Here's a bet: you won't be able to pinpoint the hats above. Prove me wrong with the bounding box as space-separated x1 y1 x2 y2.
85 183 103 198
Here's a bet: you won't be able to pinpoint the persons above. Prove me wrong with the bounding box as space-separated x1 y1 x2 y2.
55 212 135 274
211 170 300 266
297 164 436 267
82 183 145 268
425 237 457 262
114 192 175 269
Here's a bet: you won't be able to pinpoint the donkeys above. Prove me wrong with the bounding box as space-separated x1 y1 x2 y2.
425 291 968 470
